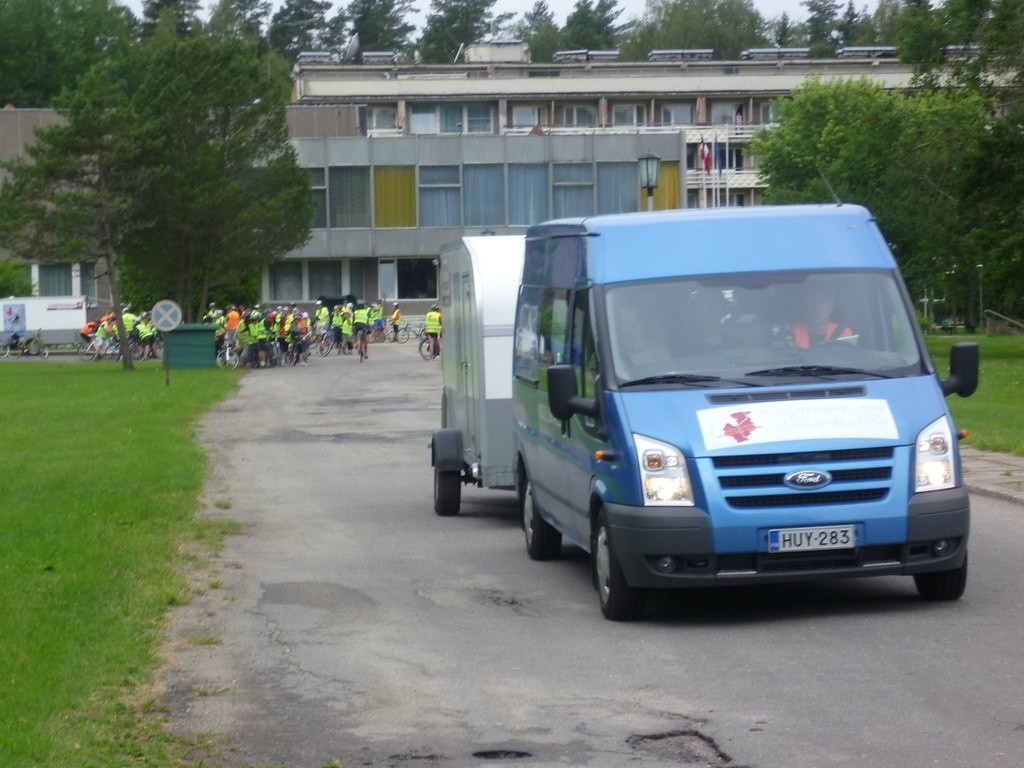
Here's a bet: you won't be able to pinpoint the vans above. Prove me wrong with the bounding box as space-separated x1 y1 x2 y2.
510 202 984 626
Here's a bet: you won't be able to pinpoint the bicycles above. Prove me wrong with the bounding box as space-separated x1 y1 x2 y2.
77 326 165 362
208 319 442 372
0 326 51 360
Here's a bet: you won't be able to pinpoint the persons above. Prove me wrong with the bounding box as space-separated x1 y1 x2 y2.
670 285 747 362
930 316 955 336
787 292 856 347
82 295 443 366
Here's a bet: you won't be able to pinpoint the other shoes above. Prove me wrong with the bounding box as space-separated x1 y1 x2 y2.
391 339 397 342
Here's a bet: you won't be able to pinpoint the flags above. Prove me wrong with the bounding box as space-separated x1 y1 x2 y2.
699 136 711 176
712 139 722 177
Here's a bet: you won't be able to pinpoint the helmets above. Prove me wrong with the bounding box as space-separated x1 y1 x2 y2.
430 303 439 311
392 303 399 309
84 299 384 326
